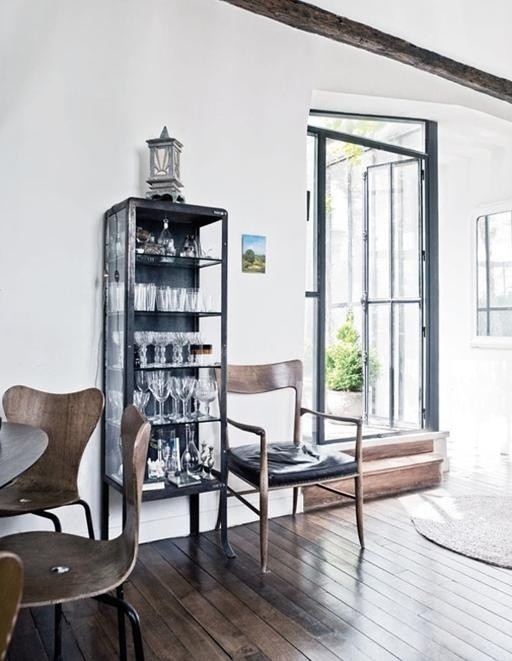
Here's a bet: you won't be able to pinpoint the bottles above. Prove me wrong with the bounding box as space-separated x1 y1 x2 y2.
133 218 205 261
111 427 219 491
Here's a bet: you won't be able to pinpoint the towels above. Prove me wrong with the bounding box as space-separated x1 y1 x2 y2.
246 441 321 464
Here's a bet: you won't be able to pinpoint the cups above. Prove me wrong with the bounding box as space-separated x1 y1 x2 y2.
112 282 216 312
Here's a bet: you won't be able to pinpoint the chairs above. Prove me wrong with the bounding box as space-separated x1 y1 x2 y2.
2 383 107 539
0 546 30 661
213 357 367 574
3 401 151 661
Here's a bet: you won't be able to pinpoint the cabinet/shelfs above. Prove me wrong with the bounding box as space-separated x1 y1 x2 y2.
97 196 239 569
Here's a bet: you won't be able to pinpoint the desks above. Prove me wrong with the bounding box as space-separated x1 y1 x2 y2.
1 419 50 498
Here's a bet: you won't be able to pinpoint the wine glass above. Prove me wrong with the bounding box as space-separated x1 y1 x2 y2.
111 369 220 425
113 331 216 367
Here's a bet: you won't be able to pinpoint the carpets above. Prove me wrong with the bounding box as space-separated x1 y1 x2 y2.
408 492 512 568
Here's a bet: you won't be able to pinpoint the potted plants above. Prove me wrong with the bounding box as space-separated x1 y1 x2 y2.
325 307 379 424
